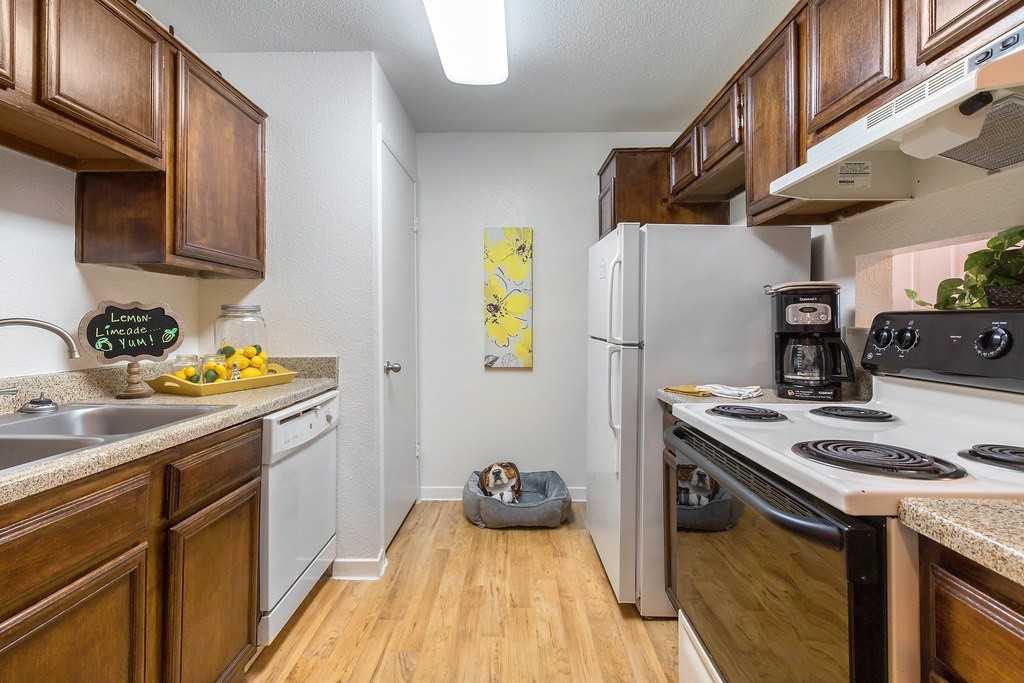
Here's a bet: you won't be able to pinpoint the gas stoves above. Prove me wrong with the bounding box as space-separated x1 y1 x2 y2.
663 307 1024 516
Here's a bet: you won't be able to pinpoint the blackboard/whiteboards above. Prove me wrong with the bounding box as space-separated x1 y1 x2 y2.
77 301 186 365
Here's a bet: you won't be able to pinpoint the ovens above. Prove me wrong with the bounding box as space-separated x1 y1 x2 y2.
663 420 920 683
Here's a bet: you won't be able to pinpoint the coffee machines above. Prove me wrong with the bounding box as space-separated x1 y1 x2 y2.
763 279 857 402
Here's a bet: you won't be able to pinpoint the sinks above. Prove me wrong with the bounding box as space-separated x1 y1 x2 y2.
0 436 123 474
0 402 238 439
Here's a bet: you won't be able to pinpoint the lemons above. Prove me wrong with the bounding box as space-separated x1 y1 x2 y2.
175 344 267 384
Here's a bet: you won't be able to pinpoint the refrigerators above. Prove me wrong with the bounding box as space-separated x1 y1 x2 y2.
584 222 813 616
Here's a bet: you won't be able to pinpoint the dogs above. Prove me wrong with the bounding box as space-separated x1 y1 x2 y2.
478 461 524 504
677 462 719 507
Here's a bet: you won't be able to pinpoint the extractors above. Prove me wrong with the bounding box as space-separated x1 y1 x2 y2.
768 22 1024 201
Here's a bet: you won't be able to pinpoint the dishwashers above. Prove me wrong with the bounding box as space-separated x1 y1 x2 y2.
258 389 339 646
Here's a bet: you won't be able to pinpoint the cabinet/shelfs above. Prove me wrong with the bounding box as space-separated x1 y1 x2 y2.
0 415 264 682
73 44 272 281
1 0 172 172
807 1 1023 162
663 403 681 612
668 71 744 205
596 146 731 238
735 6 886 230
919 534 1024 683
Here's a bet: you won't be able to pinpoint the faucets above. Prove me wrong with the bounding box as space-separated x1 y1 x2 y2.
0 318 82 360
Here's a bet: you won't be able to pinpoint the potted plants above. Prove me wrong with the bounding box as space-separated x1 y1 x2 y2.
904 223 1024 308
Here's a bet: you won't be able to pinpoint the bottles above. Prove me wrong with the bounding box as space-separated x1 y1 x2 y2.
173 355 202 385
214 303 269 381
229 363 242 380
199 355 226 384
